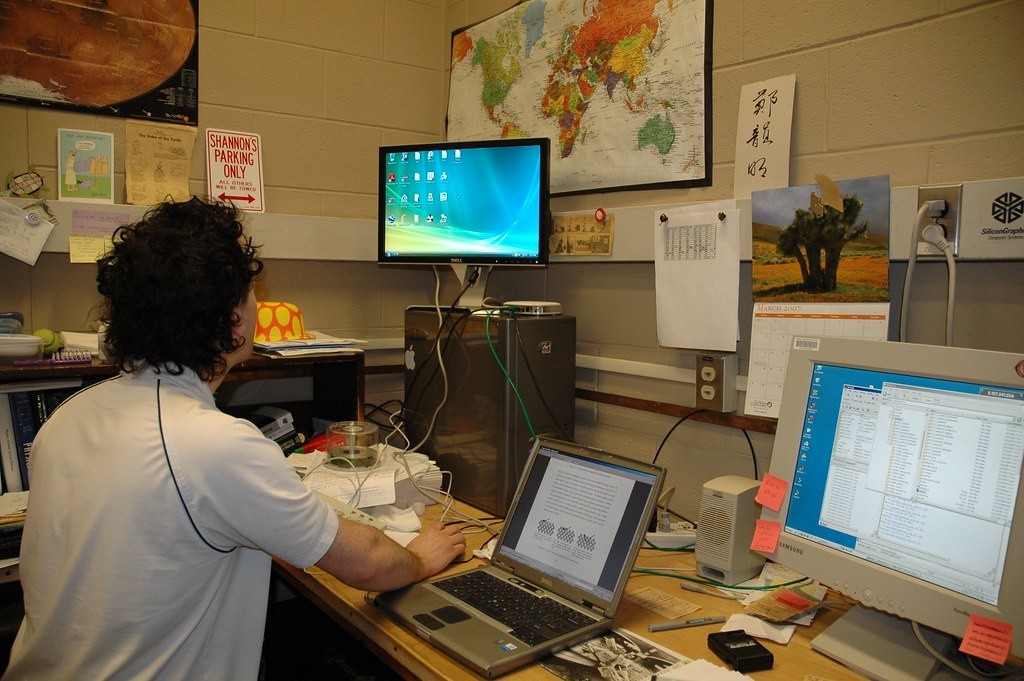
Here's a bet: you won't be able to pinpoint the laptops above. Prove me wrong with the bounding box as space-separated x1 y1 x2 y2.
374 437 668 680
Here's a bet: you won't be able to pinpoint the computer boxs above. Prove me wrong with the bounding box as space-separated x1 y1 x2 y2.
404 304 576 518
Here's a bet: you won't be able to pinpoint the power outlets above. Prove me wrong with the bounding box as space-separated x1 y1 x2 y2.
915 183 964 259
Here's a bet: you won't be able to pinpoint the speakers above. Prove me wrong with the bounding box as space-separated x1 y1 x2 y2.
696 475 766 588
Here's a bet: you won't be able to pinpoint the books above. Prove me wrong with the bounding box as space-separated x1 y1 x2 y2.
0 375 114 530
240 405 347 457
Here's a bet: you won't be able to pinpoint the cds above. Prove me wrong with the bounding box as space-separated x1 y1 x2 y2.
330 445 376 459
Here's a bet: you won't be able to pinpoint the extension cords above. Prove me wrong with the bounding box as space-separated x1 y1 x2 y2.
641 520 698 551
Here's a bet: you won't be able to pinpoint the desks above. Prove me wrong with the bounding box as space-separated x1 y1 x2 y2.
275 491 1024 681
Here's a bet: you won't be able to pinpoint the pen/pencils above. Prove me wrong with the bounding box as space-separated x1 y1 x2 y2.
647 616 726 633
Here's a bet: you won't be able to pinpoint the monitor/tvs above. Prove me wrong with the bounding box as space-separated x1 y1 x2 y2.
753 335 1024 681
378 138 551 313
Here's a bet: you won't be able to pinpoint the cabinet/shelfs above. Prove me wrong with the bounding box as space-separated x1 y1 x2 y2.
0 351 368 491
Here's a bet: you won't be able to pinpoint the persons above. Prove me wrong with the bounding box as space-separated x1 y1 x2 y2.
595 653 638 669
0 193 467 681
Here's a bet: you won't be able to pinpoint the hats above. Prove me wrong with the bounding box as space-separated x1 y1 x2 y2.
254 301 317 341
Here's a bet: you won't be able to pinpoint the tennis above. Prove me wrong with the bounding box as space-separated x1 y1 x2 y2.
31 329 60 356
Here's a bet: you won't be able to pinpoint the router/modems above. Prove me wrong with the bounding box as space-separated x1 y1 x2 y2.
500 300 562 317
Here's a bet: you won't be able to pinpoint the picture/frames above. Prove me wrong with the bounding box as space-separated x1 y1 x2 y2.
445 0 714 197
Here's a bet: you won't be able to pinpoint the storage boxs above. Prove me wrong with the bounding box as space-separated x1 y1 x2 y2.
708 628 774 673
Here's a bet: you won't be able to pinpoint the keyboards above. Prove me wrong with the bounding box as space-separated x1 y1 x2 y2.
311 489 387 534
